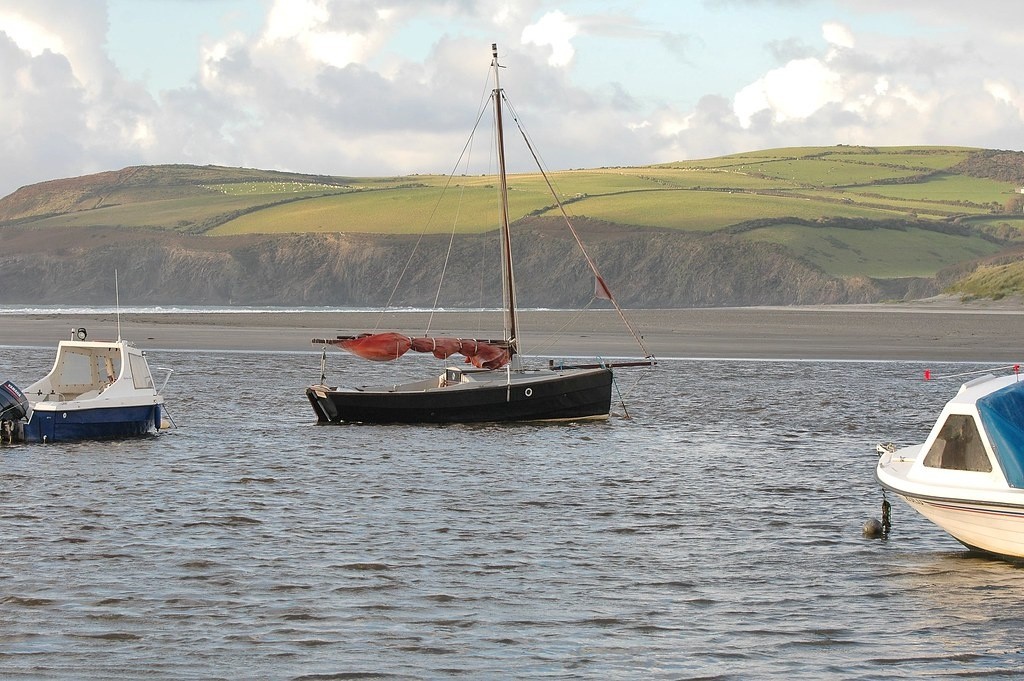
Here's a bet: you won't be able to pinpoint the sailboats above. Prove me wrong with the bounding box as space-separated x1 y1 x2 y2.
305 45 660 429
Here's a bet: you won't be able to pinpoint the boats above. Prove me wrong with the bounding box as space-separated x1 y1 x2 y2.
874 366 1024 565
0 268 174 442
